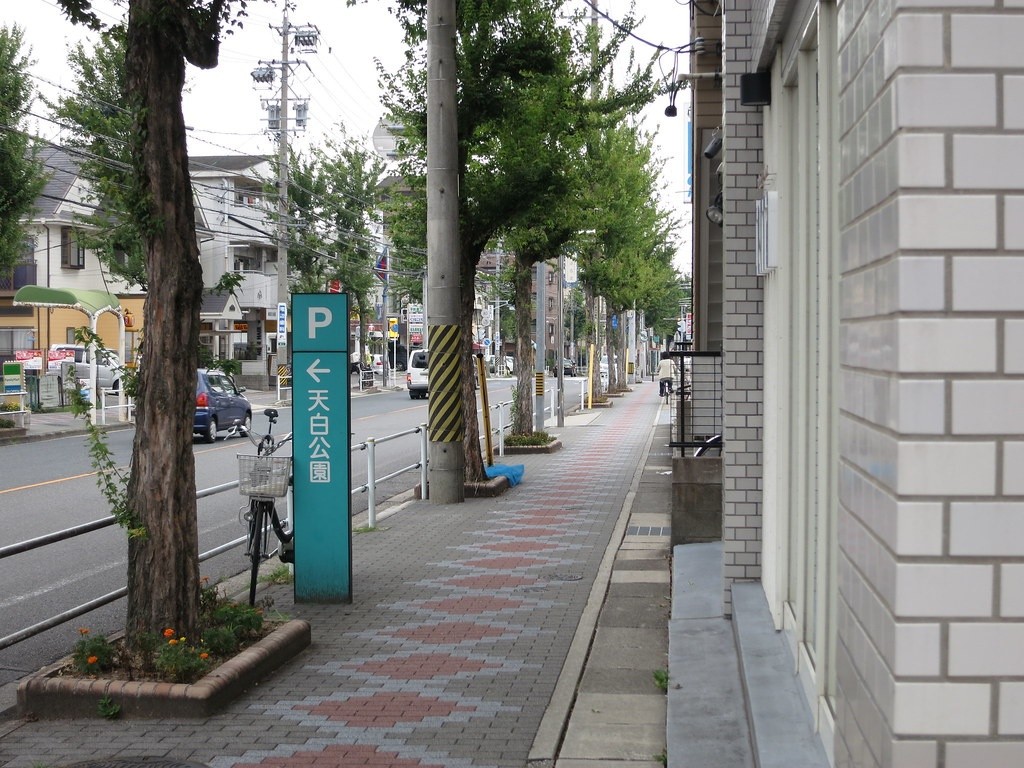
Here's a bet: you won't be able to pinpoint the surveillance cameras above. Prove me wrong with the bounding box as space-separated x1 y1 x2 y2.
704 138 722 158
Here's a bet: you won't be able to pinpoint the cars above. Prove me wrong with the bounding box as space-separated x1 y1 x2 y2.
552 356 576 376
371 353 391 379
192 369 253 441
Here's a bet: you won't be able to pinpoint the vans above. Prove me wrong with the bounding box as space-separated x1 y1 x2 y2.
51 342 124 392
405 348 429 400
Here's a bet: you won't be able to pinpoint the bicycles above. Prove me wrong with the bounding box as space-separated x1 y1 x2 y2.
664 380 670 401
226 408 293 612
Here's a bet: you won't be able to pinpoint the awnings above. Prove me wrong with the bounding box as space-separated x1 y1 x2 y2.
12 284 128 426
473 343 486 350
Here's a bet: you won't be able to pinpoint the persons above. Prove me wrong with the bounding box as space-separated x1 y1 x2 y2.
656 351 677 397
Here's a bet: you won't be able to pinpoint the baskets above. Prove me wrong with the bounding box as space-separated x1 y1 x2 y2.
236 452 292 496
